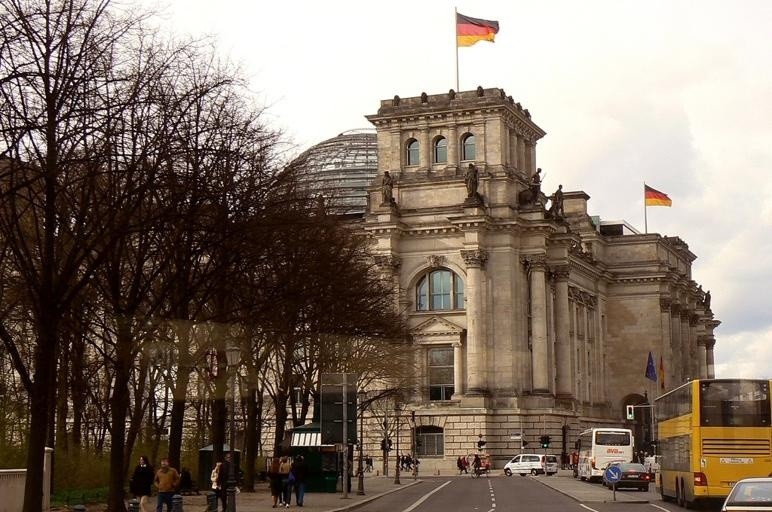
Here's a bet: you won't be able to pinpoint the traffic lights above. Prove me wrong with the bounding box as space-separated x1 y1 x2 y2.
545 435 552 444
542 444 549 448
478 441 481 448
416 437 422 451
626 404 636 419
538 436 546 443
481 441 485 446
387 439 393 452
380 439 385 450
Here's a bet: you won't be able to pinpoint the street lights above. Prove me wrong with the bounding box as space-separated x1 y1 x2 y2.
223 346 241 512
356 384 366 496
393 402 402 486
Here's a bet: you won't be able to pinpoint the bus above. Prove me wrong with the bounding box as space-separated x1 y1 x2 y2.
571 427 636 483
656 378 772 510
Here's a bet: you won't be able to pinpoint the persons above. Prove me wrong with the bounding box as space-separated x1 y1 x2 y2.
705 291 711 310
128 456 158 512
210 453 239 512
379 171 396 203
154 456 180 512
181 467 186 495
362 455 373 473
268 456 307 509
555 185 565 216
400 454 419 471
532 168 542 202
186 467 192 495
457 452 483 475
464 163 481 197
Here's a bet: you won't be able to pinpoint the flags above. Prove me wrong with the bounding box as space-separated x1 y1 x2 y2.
456 12 499 46
645 185 673 207
659 356 666 389
645 351 657 382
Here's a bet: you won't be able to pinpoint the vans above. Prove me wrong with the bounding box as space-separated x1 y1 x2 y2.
502 454 559 477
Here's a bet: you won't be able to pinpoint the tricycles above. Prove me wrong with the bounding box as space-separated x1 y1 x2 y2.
470 463 491 479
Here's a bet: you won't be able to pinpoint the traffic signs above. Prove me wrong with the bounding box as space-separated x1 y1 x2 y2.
510 436 522 440
478 448 483 452
510 432 521 436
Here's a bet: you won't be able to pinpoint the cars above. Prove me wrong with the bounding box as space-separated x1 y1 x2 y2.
602 461 627 488
608 463 651 492
643 441 656 481
720 477 772 512
